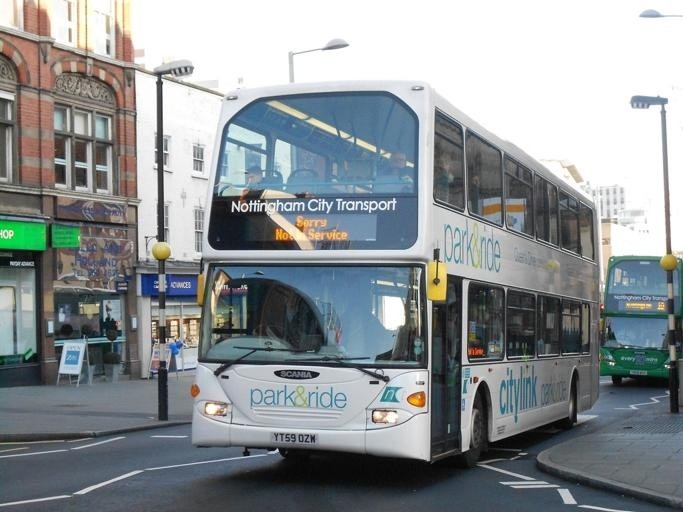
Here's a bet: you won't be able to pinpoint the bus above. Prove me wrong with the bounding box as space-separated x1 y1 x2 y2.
602 256 682 386
191 80 600 470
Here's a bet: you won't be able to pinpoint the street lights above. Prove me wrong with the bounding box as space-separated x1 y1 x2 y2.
154 60 194 420
289 39 348 83
631 96 678 412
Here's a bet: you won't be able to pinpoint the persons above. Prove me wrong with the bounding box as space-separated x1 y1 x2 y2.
372 150 414 193
237 166 270 203
436 152 462 207
270 294 309 338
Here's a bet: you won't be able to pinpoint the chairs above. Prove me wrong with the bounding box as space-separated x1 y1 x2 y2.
260 168 319 196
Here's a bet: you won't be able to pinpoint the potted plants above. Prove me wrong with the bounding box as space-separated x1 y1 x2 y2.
101 329 123 382
